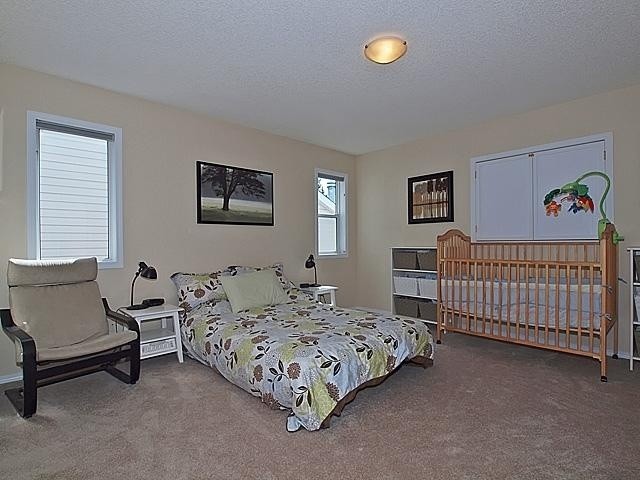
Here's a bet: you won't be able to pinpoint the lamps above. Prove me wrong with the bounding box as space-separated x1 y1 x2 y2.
305 254 321 287
364 36 407 65
127 262 157 310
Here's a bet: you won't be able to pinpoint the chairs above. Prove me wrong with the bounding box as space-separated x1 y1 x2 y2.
0 257 141 419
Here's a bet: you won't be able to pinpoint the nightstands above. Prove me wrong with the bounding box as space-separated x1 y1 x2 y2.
119 303 186 364
300 286 339 306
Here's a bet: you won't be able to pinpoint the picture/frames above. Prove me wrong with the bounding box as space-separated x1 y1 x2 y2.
408 170 453 223
196 160 274 227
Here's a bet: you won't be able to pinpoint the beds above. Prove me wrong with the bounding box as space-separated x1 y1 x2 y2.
436 223 619 382
181 309 435 432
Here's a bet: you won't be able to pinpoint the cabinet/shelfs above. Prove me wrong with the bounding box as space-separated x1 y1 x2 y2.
388 246 447 335
627 247 640 372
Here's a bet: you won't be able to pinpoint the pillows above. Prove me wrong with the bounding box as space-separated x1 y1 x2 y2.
218 268 293 313
220 264 292 292
169 268 234 311
